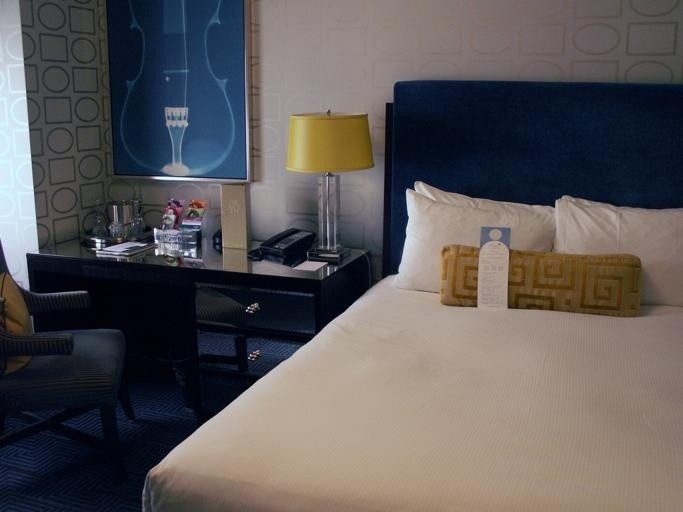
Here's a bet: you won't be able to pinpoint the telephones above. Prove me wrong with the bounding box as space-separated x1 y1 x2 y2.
259 228 315 256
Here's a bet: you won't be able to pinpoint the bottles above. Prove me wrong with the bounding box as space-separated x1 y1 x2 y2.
93 198 145 238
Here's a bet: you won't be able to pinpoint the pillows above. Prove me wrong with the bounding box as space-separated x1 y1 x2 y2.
394 180 682 318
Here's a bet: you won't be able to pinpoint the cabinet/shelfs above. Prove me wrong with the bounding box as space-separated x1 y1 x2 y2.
194 283 317 417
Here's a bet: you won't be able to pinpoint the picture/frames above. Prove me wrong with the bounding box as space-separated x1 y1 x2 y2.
107 0 254 185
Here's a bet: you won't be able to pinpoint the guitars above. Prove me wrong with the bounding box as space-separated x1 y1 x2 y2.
121 1 237 174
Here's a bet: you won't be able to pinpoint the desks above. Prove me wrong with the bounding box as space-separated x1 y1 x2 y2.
24 235 372 353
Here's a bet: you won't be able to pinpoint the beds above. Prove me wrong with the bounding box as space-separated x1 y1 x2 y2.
141 81 682 512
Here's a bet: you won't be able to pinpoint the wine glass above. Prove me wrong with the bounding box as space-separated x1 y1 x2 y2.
162 107 190 176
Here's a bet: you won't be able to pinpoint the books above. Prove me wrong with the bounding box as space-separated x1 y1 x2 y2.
94 239 158 257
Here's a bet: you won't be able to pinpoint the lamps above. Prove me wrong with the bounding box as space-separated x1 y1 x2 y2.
286 108 375 264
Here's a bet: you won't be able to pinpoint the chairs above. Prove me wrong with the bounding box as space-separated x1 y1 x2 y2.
1 237 135 451
